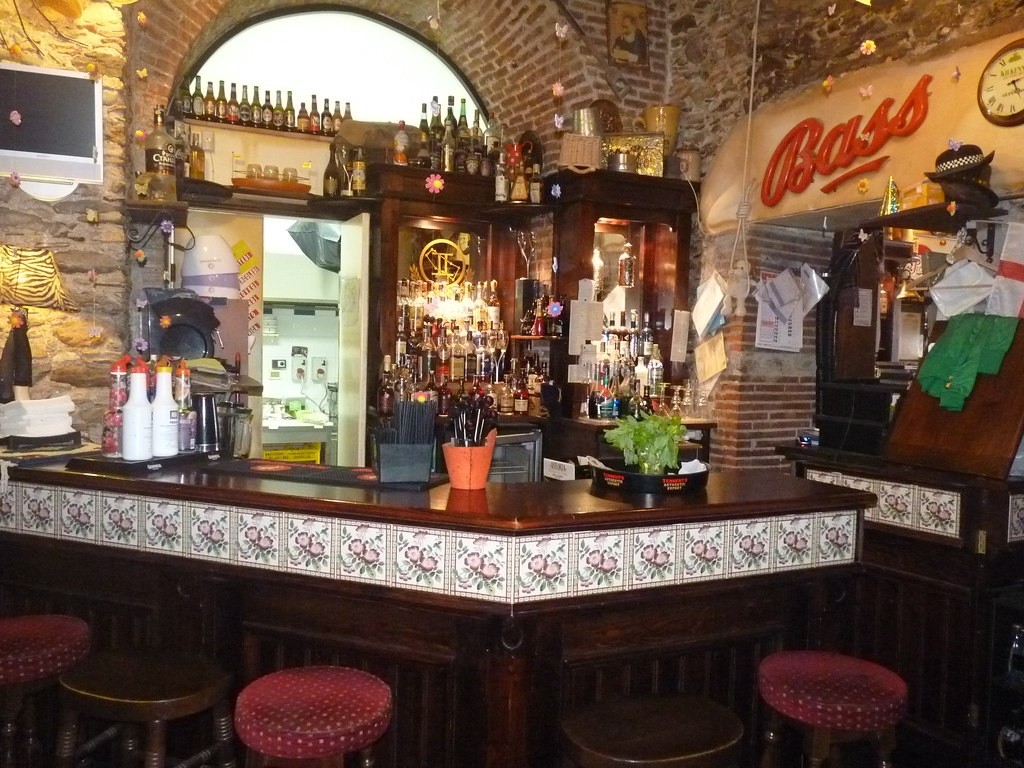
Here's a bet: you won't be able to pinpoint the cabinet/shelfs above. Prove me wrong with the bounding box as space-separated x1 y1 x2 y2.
777 434 1024 768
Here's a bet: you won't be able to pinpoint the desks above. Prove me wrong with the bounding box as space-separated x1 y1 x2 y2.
528 413 718 481
262 404 334 465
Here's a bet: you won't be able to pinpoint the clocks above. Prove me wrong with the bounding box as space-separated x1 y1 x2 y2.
977 38 1024 127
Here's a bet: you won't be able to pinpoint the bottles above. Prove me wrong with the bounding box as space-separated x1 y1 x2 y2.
171 74 352 137
101 354 198 462
418 96 545 205
378 278 564 417
618 243 635 287
215 401 254 459
592 247 604 290
393 121 409 166
323 142 368 197
580 308 664 421
145 103 205 204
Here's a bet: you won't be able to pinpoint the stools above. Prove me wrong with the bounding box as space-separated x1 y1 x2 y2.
235 665 394 768
558 691 744 768
55 645 238 768
757 650 909 768
0 612 92 768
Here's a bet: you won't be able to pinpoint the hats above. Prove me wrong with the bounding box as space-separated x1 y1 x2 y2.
922 144 1000 181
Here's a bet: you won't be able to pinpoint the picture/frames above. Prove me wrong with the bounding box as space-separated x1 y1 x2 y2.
605 0 651 72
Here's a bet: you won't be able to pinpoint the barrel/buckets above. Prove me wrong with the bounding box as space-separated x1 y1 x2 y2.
446 487 489 514
442 442 494 491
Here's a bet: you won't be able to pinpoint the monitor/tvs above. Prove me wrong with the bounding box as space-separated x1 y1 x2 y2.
0 61 104 184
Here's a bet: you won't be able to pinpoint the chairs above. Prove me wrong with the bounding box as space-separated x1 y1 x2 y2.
482 428 543 484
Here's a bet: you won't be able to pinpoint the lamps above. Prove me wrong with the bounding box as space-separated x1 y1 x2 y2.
0 244 80 330
261 316 279 337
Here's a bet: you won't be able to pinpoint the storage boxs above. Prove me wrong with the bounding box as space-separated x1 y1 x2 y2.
374 436 434 485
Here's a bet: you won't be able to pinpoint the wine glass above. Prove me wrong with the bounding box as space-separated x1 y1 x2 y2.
518 230 538 280
656 383 716 419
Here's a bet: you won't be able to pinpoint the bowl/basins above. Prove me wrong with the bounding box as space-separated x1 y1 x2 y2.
571 107 605 138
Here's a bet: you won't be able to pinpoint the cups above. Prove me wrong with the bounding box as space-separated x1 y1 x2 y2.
608 153 636 173
247 163 298 184
672 142 708 182
193 393 224 452
632 105 682 157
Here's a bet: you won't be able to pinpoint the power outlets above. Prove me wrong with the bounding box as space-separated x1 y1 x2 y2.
292 356 307 383
311 357 328 384
